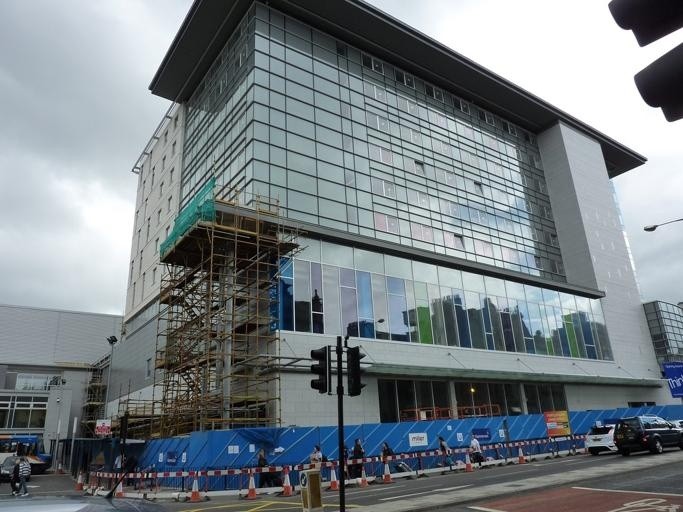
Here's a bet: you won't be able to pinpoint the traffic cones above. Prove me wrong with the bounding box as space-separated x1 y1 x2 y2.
75 470 83 491
57 460 66 474
358 464 369 488
327 463 339 491
115 473 124 498
243 471 261 500
281 467 296 497
464 450 475 472
516 444 527 464
380 459 394 484
187 474 203 503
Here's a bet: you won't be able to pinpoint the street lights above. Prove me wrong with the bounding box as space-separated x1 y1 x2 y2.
642 217 683 232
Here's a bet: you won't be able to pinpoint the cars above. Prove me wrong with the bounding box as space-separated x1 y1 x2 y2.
0 456 30 483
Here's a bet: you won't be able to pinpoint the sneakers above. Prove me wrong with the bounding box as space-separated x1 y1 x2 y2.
10 491 29 496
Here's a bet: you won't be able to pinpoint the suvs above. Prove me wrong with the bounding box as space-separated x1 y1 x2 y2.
584 415 682 455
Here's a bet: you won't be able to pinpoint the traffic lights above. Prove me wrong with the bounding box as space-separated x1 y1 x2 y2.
309 345 331 395
606 0 681 121
345 346 369 397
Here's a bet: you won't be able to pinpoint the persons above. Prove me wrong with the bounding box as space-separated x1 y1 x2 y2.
112 452 124 485
353 439 365 478
8 442 32 497
382 441 393 473
95 450 105 487
344 440 349 479
470 435 481 463
125 455 137 487
439 436 456 466
309 445 323 463
258 448 269 488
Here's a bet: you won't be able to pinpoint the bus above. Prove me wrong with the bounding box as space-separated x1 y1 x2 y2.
0 434 52 475
